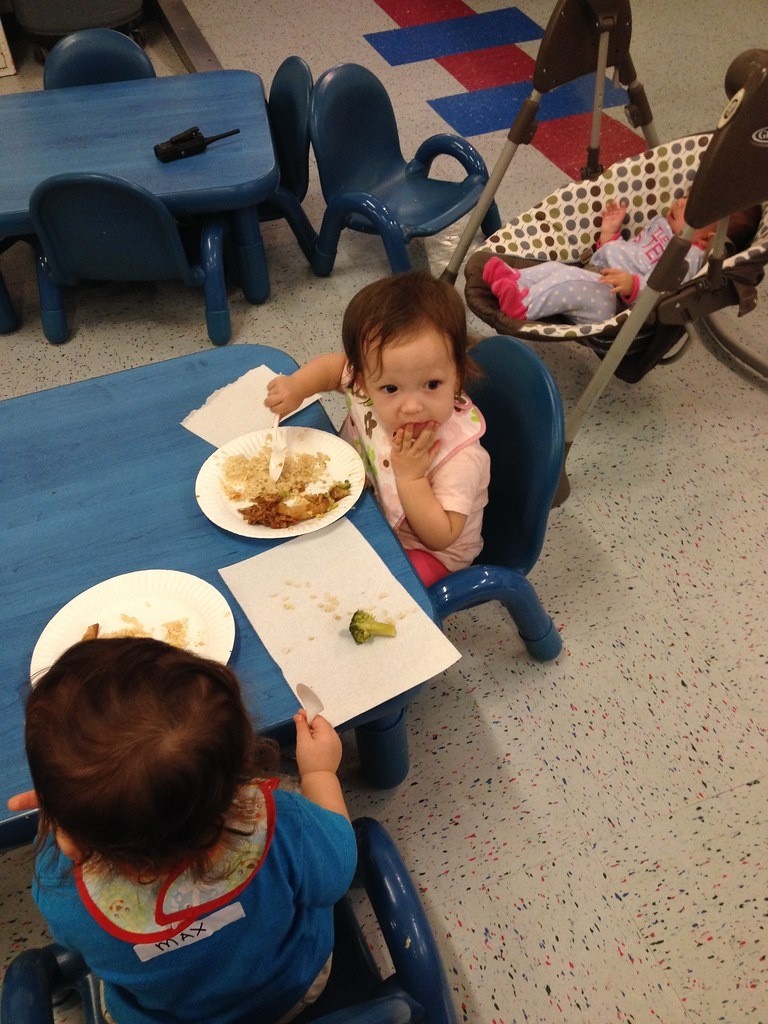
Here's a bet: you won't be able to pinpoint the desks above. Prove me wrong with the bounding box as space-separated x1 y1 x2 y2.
0 342 443 856
0 69 281 336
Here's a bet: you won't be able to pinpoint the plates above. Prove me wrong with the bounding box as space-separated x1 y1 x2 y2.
195 426 366 538
30 569 236 688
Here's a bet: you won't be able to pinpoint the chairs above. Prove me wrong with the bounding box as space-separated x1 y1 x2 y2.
0 816 458 1024
174 56 318 288
353 335 566 793
29 171 232 347
308 61 503 278
43 28 156 90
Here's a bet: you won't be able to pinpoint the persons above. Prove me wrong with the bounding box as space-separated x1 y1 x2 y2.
264 272 490 588
482 190 763 324
23 636 358 1024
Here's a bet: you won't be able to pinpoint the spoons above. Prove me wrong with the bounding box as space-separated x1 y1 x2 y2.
297 684 325 726
269 372 285 482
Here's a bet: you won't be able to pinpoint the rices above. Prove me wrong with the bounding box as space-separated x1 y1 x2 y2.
219 435 329 502
98 613 190 649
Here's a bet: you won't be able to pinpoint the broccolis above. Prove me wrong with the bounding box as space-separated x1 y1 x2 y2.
349 610 396 645
